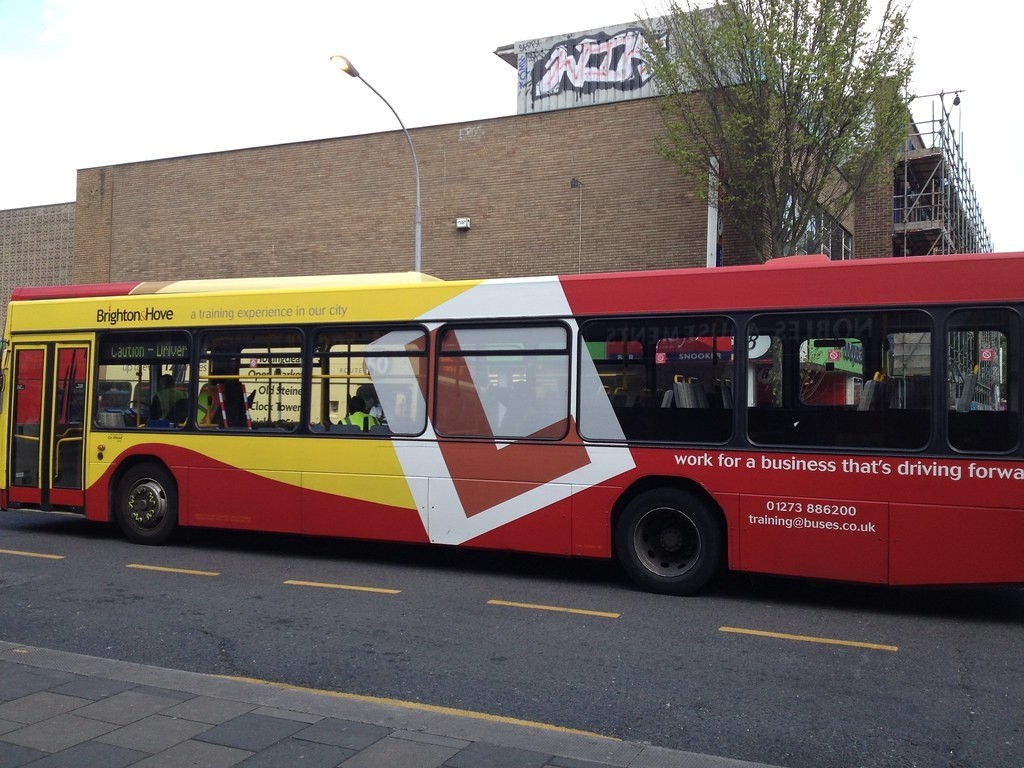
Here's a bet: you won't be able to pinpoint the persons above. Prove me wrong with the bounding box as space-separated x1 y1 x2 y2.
147 374 190 421
195 346 252 431
56 387 63 418
338 384 387 433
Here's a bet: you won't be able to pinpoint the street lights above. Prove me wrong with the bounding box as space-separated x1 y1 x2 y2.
329 55 422 271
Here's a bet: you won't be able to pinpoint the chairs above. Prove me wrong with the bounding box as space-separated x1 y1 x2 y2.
957 366 979 414
660 373 734 409
165 398 189 429
857 372 892 411
599 386 663 407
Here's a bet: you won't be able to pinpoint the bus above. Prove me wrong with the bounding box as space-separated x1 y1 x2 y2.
0 252 1024 597
